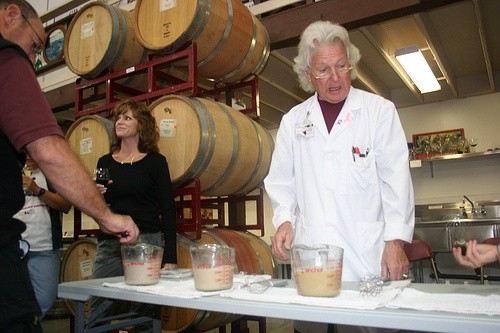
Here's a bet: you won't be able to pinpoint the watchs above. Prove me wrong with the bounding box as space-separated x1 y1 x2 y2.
38 187 45 197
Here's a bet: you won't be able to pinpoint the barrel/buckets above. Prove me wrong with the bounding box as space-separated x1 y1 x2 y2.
132 0 272 84
59 227 278 333
147 95 275 198
64 115 116 178
63 0 189 97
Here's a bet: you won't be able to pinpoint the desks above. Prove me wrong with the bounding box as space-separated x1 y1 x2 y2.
57 267 500 333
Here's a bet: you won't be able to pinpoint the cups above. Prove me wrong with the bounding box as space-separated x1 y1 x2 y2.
290 243 342 298
189 244 235 291
120 242 164 286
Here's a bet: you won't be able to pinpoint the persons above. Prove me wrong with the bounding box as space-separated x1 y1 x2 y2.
451 238 500 268
0 0 140 333
263 20 414 333
85 98 179 333
13 152 74 324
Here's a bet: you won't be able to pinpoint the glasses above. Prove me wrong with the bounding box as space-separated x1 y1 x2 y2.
308 63 353 81
21 12 44 53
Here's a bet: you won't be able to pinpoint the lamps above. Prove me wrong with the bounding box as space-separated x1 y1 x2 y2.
395 45 442 94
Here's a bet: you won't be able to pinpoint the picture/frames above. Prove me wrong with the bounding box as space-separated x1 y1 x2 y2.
412 128 465 159
43 24 66 64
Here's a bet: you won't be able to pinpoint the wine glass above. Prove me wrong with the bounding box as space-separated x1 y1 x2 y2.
408 136 477 159
93 168 111 208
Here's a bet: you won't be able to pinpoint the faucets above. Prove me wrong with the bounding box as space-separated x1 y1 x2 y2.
462 195 475 213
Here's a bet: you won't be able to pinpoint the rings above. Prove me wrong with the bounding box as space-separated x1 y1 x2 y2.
403 273 408 279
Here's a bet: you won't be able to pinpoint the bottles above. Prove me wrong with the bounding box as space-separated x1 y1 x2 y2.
22 161 33 196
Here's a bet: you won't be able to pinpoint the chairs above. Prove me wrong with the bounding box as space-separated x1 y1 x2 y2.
404 240 438 283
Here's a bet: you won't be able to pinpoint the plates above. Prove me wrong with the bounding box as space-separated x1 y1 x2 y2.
161 269 192 279
233 275 271 284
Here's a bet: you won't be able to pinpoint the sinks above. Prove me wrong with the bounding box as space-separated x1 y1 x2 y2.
413 220 451 253
447 218 500 253
